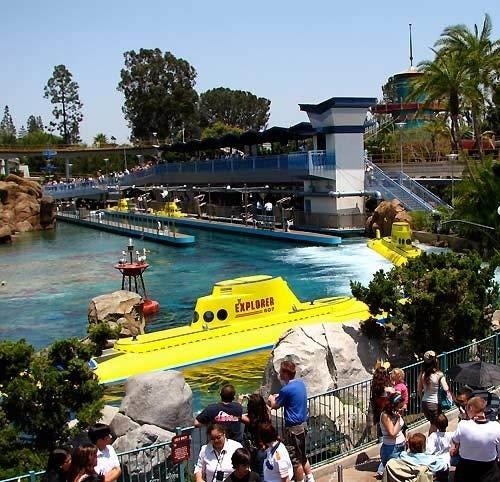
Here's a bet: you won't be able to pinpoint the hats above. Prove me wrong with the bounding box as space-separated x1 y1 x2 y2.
424 350 436 364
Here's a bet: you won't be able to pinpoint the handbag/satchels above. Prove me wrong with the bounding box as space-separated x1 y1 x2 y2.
438 385 454 410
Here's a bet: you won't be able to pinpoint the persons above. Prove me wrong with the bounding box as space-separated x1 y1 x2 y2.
194 385 293 482
261 200 273 226
268 360 315 482
256 199 263 222
370 362 408 468
417 350 448 436
449 387 499 482
41 423 121 482
396 433 450 474
45 143 308 190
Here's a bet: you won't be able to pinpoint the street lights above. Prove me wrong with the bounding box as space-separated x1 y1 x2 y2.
68 163 74 180
444 153 459 227
136 154 143 164
103 158 110 175
395 122 409 183
